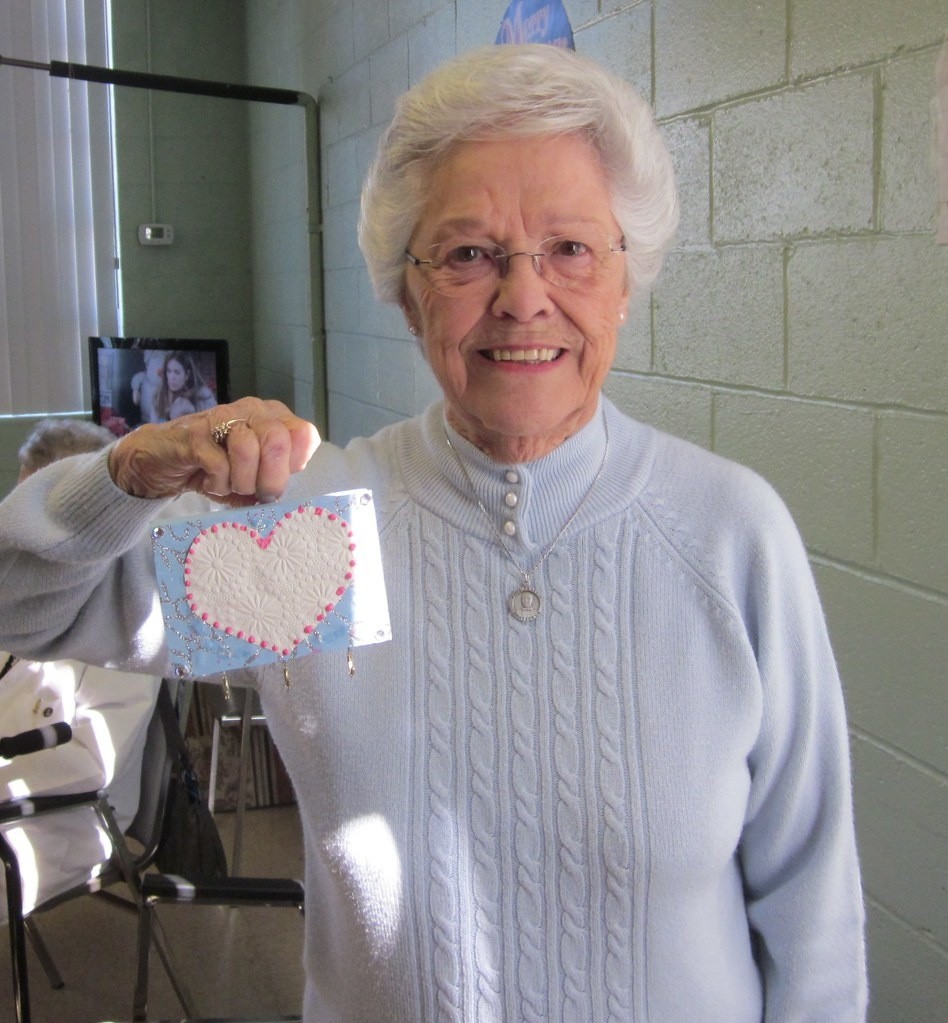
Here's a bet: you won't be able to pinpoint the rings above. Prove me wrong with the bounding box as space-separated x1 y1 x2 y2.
211 417 247 442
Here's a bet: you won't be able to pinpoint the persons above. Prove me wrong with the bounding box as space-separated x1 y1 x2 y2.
2 37 869 1023
153 348 218 426
16 417 120 485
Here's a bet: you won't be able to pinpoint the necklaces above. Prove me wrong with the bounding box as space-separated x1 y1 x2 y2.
443 401 609 623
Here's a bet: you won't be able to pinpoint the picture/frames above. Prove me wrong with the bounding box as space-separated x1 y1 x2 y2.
87 335 230 442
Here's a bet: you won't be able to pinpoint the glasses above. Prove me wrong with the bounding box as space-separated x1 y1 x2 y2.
403 231 628 299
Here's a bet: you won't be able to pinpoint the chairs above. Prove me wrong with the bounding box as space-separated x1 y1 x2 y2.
0 673 201 1022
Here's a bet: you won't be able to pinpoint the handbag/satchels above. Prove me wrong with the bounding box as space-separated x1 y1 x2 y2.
152 779 228 877
